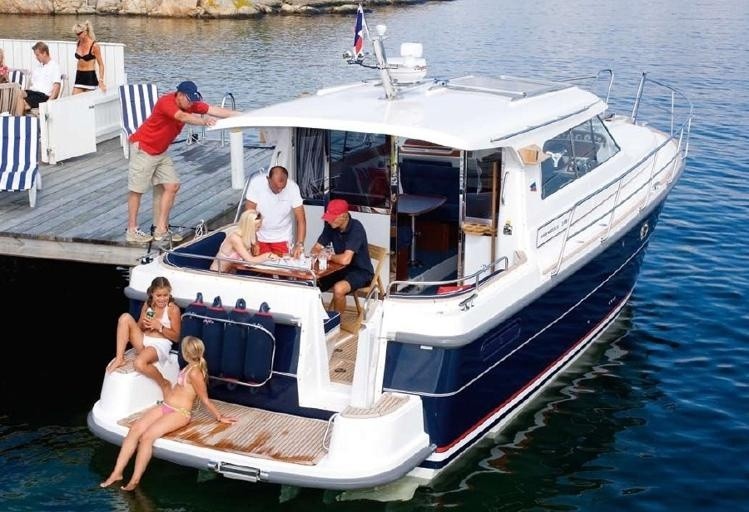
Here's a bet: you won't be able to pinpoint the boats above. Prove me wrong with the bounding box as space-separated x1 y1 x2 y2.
80 0 699 496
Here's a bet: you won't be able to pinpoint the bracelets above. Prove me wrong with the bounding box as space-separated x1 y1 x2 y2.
159 324 164 332
100 78 104 82
219 415 222 423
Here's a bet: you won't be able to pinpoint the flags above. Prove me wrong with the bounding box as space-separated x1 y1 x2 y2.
352 5 364 59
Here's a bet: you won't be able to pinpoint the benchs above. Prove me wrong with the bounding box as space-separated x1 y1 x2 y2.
337 153 561 222
167 232 228 271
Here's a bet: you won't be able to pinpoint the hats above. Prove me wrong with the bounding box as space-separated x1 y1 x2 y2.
176 81 203 102
321 199 350 223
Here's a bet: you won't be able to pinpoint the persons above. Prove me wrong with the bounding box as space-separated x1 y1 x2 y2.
72 20 107 94
309 198 374 328
106 277 181 403
245 166 307 281
100 336 240 491
15 43 61 117
366 160 425 268
210 210 280 273
125 80 242 244
0 49 10 83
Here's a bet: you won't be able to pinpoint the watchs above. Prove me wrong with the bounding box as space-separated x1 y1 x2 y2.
296 241 306 252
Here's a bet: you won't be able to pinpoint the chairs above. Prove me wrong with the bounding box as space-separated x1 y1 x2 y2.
0 114 43 209
115 82 161 161
1 67 70 116
327 241 387 336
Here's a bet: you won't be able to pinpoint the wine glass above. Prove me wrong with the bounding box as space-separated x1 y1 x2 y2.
285 240 295 256
304 249 318 272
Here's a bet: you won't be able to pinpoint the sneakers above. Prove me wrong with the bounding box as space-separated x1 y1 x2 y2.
152 229 183 242
124 227 153 242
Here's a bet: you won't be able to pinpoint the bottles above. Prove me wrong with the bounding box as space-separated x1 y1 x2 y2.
317 249 328 270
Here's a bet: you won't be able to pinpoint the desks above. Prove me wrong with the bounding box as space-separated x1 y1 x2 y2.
395 193 449 268
236 253 348 284
